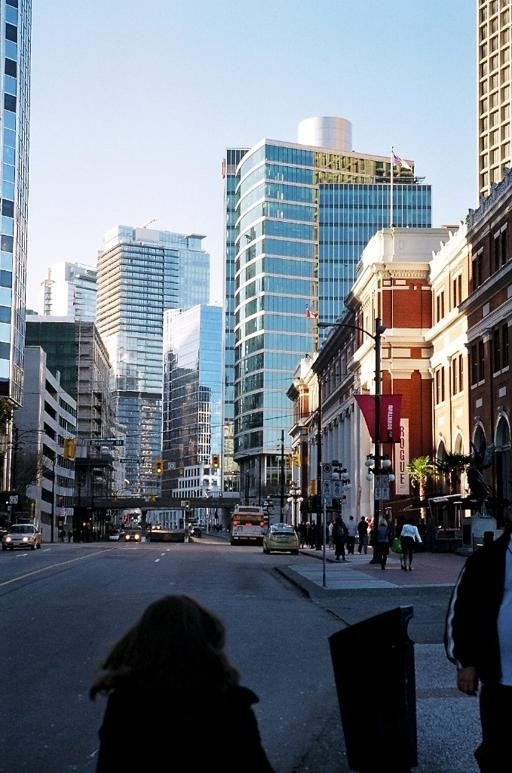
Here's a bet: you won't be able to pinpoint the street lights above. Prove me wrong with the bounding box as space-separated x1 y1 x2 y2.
317 318 383 564
11 428 46 524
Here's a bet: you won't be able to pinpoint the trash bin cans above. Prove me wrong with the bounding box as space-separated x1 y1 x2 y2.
327 604 419 773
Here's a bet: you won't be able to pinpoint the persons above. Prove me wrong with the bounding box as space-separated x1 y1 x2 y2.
59 527 103 543
88 593 279 771
297 511 439 572
443 511 512 772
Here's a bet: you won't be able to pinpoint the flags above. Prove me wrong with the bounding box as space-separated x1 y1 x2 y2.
354 392 376 443
379 393 403 442
391 147 412 170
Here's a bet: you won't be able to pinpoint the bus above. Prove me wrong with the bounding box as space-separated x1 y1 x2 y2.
231 504 264 544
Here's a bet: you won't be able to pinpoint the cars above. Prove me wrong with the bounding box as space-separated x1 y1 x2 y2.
190 528 201 538
109 524 162 541
262 523 300 555
2 524 42 550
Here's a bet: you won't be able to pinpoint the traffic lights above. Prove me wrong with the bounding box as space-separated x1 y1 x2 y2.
212 454 220 468
156 460 163 476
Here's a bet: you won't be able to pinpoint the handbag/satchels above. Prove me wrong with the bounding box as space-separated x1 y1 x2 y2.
393 538 403 553
